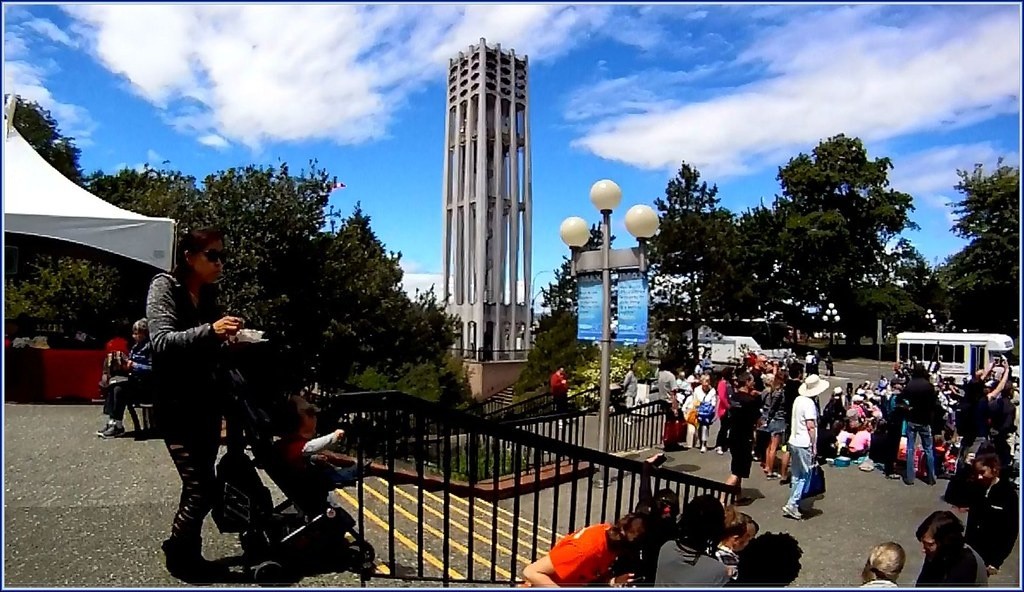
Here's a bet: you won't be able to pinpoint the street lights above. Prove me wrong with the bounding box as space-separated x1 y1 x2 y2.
822 303 841 351
924 309 937 332
559 180 659 488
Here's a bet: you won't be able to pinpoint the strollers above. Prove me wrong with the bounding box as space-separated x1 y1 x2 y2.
210 340 375 589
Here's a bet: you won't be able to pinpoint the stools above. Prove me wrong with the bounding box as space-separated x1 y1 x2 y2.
128 401 154 435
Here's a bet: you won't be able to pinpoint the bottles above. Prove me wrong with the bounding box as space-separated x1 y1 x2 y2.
59 319 64 333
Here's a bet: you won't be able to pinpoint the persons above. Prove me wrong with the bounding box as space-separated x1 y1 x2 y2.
96 318 153 438
549 366 569 429
915 511 988 589
146 228 253 586
657 349 1019 520
962 453 1019 577
270 396 359 496
523 453 804 588
623 363 637 425
857 541 906 587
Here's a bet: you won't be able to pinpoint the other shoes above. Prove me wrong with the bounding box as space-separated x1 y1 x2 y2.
715 446 723 455
162 537 229 579
782 503 802 520
752 450 791 486
96 423 114 436
700 446 707 453
833 451 956 486
101 424 125 438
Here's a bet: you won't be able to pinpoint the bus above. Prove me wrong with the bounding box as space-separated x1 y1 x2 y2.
895 331 1015 386
658 317 796 370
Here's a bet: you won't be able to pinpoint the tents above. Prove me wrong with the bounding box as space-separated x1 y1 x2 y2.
4 94 176 273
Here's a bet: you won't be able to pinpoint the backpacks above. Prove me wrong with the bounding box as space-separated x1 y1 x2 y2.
955 400 979 436
211 442 273 536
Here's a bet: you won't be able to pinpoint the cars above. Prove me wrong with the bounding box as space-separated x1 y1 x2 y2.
796 329 847 341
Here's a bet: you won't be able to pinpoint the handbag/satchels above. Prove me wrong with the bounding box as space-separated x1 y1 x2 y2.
664 415 687 443
687 401 700 430
898 436 907 461
698 397 715 425
801 457 826 501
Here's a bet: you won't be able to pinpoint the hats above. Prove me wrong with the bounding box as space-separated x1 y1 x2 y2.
798 374 830 398
833 379 902 420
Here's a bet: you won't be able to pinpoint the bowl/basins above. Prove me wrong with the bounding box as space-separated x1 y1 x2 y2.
236 329 265 341
835 458 850 467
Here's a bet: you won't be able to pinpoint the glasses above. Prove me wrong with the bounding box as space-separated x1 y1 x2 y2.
201 248 227 264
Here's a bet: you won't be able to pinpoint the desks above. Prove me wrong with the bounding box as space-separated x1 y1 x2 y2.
5 347 104 403
